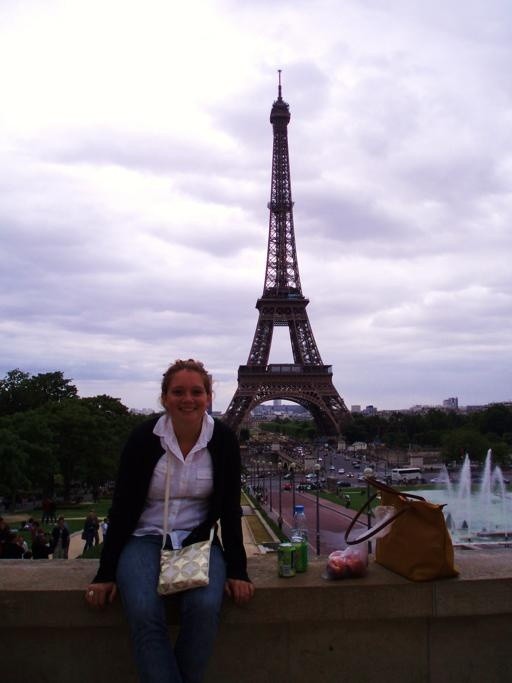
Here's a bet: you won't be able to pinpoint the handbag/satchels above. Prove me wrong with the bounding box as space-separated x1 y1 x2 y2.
156 542 212 594
373 488 461 582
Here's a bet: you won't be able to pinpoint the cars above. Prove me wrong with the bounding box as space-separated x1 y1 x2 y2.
268 431 364 488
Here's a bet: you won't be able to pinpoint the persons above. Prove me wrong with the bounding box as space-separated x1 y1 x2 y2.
0 477 116 561
81 357 257 683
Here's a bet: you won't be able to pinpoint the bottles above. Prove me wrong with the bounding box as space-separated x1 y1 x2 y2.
290 506 308 544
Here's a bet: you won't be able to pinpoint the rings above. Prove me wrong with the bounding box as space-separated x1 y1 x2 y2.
88 590 94 597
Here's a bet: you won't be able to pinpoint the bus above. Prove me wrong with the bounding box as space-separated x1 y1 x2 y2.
389 466 423 481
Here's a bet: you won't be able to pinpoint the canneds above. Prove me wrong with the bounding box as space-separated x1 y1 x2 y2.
277 543 297 577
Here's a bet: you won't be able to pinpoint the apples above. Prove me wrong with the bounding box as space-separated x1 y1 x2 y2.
326 549 368 580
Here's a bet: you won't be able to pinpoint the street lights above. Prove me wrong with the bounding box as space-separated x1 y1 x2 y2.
248 446 324 558
362 466 375 554
